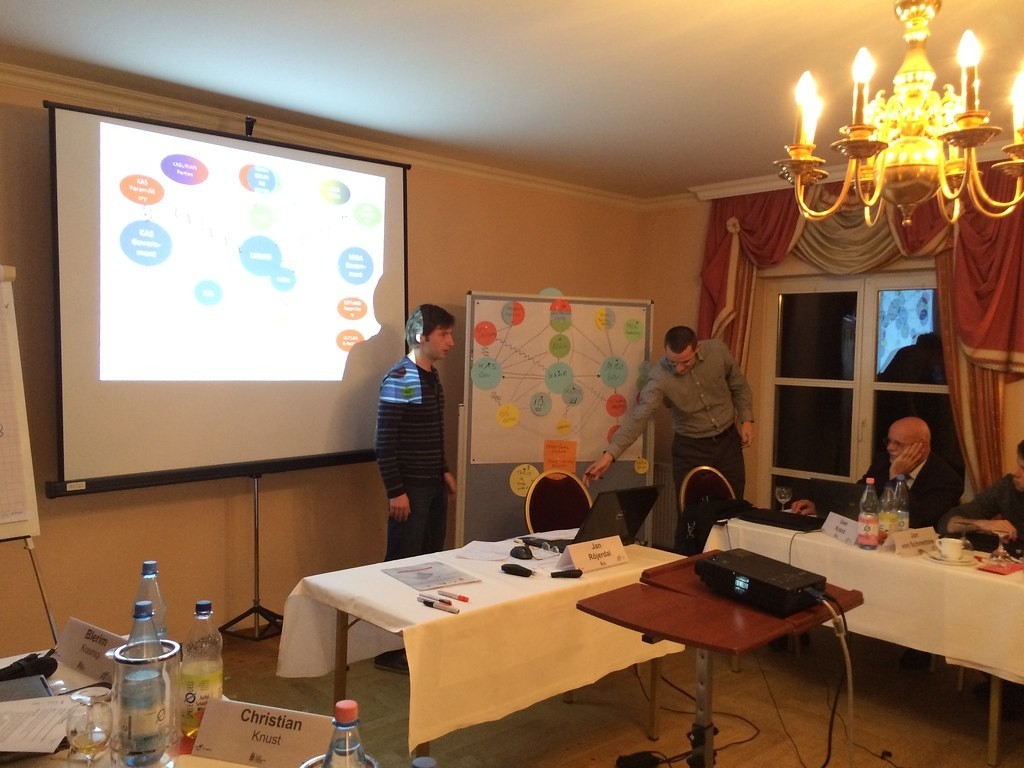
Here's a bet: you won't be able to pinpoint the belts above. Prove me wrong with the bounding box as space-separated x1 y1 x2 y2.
709 422 736 440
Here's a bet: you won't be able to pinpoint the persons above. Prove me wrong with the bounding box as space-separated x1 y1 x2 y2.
584 326 753 513
939 440 1024 723
877 333 942 452
373 304 459 673
791 417 962 672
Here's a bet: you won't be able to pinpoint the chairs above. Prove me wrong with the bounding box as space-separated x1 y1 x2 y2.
680 464 736 522
524 469 592 535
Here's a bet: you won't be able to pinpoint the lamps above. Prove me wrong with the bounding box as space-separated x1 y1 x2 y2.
772 0 1024 227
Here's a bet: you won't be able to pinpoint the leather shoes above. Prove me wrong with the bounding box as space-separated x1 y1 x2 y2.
374 651 410 674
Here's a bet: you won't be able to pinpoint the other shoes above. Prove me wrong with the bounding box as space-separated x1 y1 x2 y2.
898 646 931 670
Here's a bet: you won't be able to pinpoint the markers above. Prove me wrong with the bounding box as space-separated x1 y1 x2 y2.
974 555 1006 568
584 473 604 480
437 590 469 602
423 600 459 614
797 507 803 514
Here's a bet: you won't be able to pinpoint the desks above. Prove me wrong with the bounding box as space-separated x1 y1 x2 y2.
575 549 864 766
277 527 690 756
702 510 1023 767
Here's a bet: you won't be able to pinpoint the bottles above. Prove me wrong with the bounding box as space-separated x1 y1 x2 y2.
878 482 898 545
181 600 222 740
320 699 366 768
132 564 166 642
120 601 167 768
894 475 909 531
857 478 879 550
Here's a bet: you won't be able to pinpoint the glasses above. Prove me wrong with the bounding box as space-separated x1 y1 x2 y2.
666 361 691 367
882 435 923 449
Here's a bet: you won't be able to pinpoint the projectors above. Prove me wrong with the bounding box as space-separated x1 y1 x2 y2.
694 549 826 616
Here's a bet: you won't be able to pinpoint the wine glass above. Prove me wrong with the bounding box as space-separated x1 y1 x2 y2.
776 487 792 511
955 521 975 554
991 528 1012 561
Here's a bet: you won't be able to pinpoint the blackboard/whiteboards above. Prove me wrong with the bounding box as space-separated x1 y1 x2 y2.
465 291 653 461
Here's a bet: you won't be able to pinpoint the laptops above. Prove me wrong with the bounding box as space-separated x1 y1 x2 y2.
523 484 665 554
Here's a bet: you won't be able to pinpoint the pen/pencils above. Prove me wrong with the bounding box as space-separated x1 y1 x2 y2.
418 592 452 604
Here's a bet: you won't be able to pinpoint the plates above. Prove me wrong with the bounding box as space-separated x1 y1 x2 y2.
921 552 977 566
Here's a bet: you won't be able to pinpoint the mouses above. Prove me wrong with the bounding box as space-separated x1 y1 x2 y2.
511 546 536 559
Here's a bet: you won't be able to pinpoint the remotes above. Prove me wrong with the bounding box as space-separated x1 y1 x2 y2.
552 570 583 578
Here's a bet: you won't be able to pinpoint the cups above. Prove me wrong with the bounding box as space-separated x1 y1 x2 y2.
112 639 180 768
67 687 113 756
298 753 379 768
935 538 963 559
